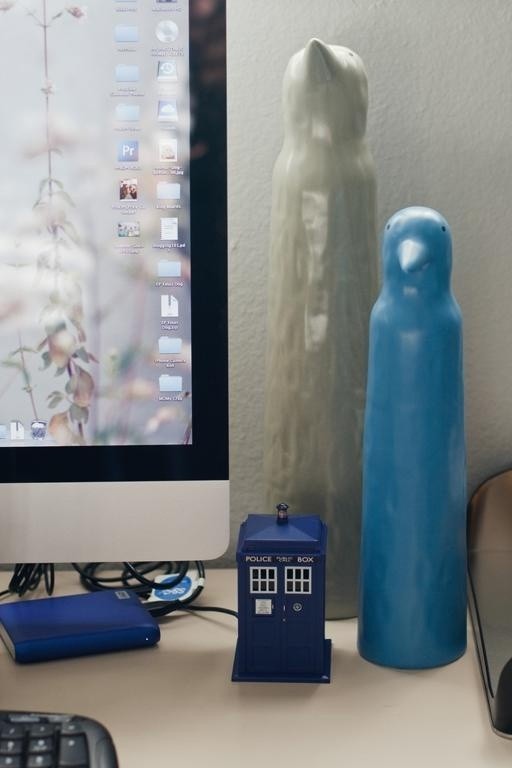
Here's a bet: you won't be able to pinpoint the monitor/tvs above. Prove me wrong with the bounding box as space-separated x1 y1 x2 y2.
0 0 232 562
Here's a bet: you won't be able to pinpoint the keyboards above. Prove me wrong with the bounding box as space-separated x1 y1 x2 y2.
1 705 121 767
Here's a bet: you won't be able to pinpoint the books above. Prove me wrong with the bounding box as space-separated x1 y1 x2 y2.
0 588 161 664
